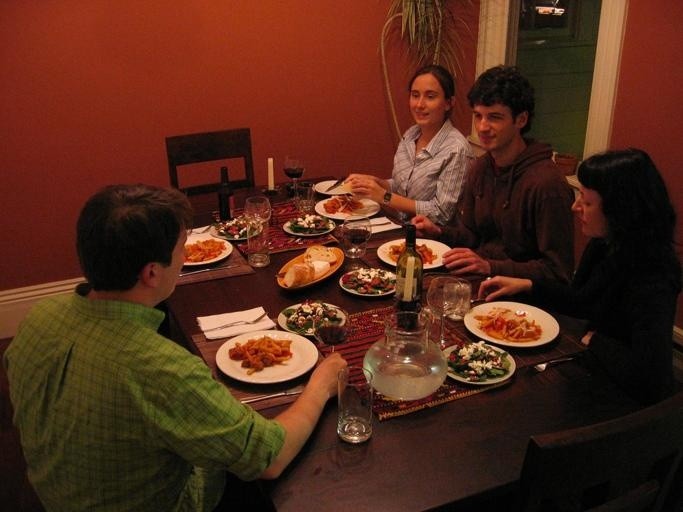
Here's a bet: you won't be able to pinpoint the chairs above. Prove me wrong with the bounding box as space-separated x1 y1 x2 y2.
165 128 255 196
517 392 683 512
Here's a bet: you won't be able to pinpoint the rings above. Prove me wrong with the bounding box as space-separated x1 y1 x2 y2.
487 276 491 280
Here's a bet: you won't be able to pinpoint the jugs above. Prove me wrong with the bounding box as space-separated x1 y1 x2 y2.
363 311 449 400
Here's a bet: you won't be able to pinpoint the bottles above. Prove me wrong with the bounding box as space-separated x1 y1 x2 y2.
396 224 425 329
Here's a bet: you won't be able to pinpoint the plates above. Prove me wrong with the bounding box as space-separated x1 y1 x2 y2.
182 234 233 266
210 222 264 241
217 329 319 387
378 238 451 270
315 180 348 194
338 268 396 299
465 302 561 348
276 247 345 290
278 303 348 337
443 341 518 386
281 220 337 237
315 197 382 221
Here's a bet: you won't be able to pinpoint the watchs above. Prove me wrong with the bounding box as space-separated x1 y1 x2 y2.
382 191 392 209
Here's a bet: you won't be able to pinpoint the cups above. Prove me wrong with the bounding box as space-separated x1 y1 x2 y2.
443 279 472 321
338 226 368 274
345 215 372 240
335 364 374 443
246 218 271 268
295 182 316 216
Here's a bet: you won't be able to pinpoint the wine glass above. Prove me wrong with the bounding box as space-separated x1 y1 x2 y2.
426 277 463 347
284 156 306 196
245 196 273 253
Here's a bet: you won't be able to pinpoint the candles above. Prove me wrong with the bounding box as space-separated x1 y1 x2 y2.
268 156 274 190
404 255 415 302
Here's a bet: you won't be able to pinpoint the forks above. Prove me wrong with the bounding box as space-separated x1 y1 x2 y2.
534 357 577 373
203 313 267 335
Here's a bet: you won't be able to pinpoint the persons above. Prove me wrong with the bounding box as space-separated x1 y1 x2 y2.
478 146 683 434
0 182 348 512
343 65 476 225
409 65 576 284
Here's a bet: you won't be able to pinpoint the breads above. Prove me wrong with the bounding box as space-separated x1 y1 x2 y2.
282 244 339 288
